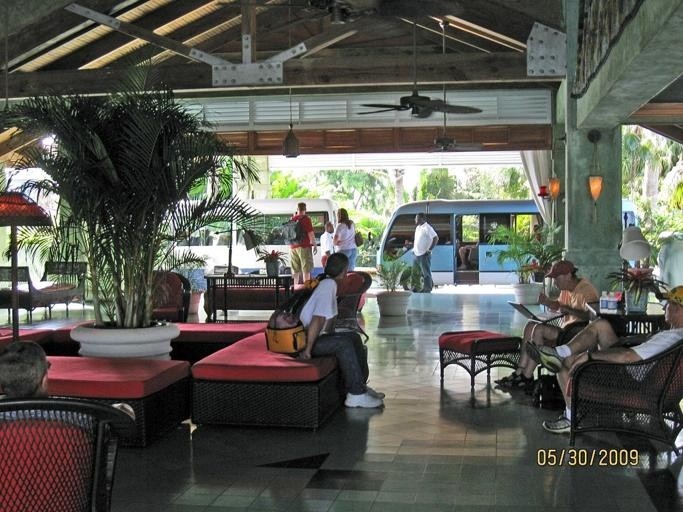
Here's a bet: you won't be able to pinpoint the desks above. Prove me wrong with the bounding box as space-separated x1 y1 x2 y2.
205 274 295 323
593 301 665 338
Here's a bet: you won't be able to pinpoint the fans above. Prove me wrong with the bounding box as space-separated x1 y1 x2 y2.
354 11 497 156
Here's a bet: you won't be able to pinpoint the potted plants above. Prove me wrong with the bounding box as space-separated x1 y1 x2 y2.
487 220 566 306
0 47 269 360
366 261 422 316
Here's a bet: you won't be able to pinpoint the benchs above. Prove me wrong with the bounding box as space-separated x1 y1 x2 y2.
2 321 268 359
191 321 346 435
204 283 306 323
41 355 189 448
0 259 86 323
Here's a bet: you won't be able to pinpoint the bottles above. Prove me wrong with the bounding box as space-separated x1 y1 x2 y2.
607 292 618 315
599 290 610 314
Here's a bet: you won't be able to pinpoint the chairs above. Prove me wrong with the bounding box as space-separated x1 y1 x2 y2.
537 300 601 379
291 270 373 344
0 397 140 512
568 339 683 457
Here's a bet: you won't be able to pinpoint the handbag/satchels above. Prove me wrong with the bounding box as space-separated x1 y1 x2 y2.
355 232 363 246
532 375 579 411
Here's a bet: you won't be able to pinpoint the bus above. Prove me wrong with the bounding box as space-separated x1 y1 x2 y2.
117 199 342 292
367 199 637 287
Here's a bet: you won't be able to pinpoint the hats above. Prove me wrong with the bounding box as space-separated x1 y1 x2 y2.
656 286 683 305
545 262 573 278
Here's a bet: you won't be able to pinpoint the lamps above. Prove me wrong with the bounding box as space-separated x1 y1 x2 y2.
0 190 54 341
282 87 300 158
537 185 551 201
619 223 651 261
548 134 566 202
586 127 603 225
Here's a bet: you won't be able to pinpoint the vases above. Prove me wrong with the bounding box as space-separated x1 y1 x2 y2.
624 287 649 312
266 261 280 276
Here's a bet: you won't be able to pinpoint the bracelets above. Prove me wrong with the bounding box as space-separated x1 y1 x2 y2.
587 349 593 360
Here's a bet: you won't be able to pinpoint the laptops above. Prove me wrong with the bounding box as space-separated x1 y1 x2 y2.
507 301 568 324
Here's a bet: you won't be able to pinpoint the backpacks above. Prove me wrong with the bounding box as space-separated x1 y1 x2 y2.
282 215 306 245
265 274 331 355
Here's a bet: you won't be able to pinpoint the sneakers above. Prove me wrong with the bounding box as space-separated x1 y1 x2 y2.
525 341 564 372
543 418 571 433
344 387 385 408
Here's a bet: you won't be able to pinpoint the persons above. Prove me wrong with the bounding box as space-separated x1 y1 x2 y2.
533 224 541 241
320 221 334 272
1 339 52 397
333 207 359 272
525 287 683 435
412 213 439 293
290 203 317 284
284 252 385 408
494 260 600 392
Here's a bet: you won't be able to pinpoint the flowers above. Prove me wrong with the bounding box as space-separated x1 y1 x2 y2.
256 248 290 267
604 260 670 306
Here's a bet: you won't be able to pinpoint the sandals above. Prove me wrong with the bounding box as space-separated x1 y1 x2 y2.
495 372 534 389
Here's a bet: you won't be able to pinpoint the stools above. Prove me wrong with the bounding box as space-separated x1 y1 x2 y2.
439 330 522 389
